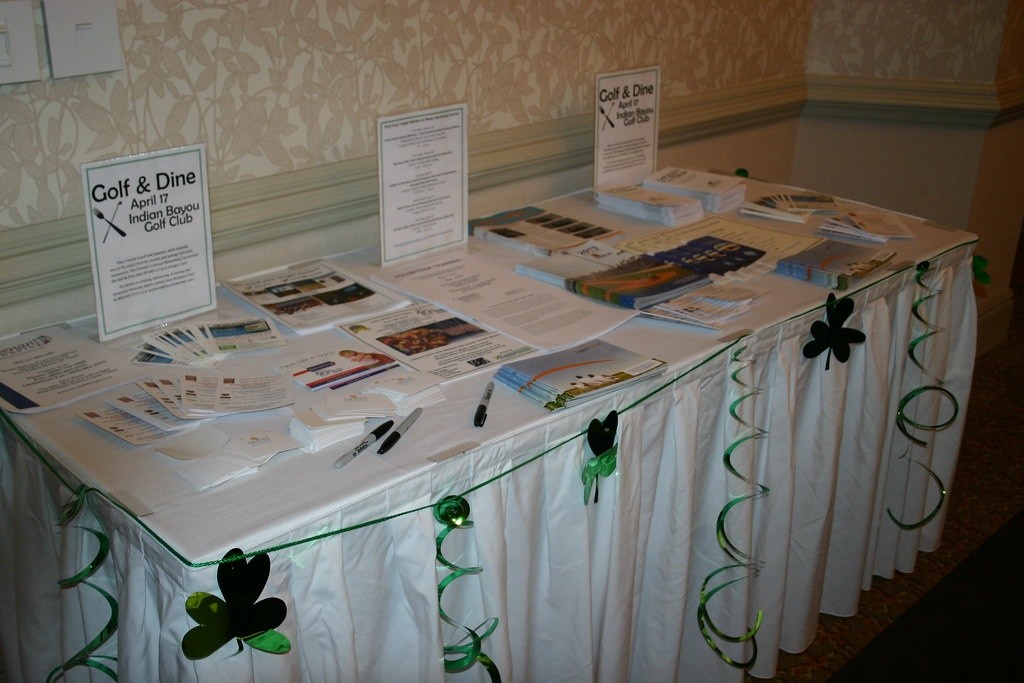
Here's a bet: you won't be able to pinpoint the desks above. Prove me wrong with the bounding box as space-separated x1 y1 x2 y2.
0 169 981 683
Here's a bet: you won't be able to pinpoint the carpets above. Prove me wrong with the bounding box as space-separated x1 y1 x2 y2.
827 508 1024 683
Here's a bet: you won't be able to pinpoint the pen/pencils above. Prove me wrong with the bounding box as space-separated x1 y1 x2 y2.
333 420 394 470
376 406 423 454
473 381 495 428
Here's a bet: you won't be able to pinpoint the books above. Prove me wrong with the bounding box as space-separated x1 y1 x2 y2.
80 249 664 496
0 321 152 417
596 166 748 231
464 204 788 332
736 182 921 291
